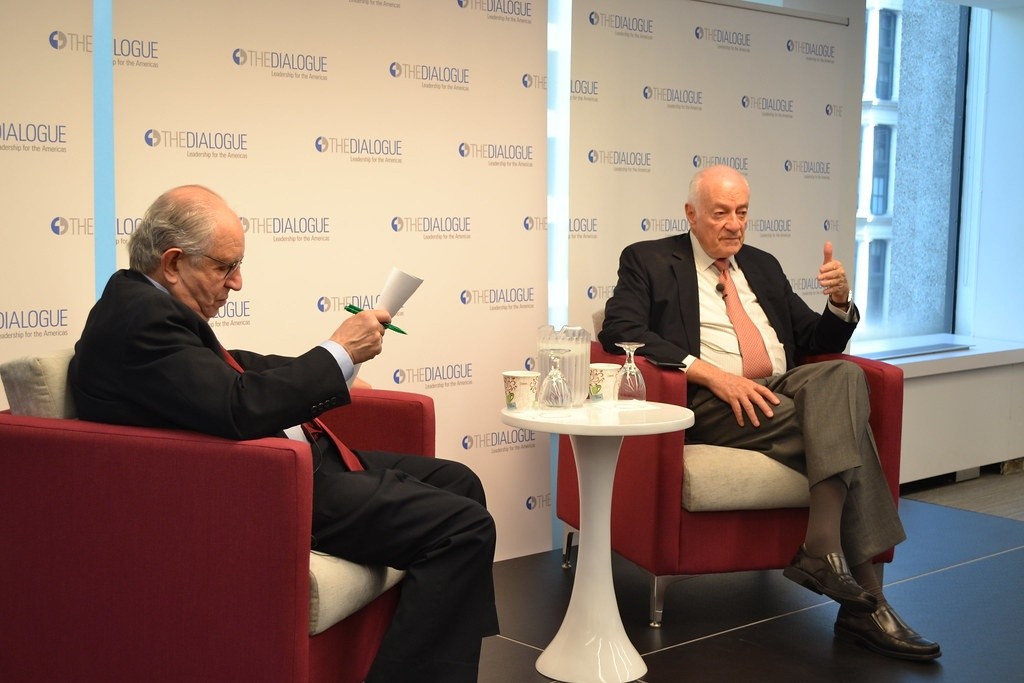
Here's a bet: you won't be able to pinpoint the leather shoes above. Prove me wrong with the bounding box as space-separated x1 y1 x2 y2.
834 602 942 660
783 543 878 609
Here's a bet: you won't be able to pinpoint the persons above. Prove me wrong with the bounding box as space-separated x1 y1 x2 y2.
66 184 501 683
599 164 944 667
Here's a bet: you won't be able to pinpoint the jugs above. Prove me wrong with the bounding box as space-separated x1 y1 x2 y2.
534 325 592 406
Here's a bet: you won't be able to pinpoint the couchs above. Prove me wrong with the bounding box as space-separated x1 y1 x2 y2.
554 308 905 631
0 348 436 683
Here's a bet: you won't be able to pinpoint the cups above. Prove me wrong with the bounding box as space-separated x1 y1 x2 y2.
502 370 541 410
589 363 622 400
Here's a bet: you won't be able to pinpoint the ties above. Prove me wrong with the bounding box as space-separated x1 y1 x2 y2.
712 258 772 380
217 342 364 472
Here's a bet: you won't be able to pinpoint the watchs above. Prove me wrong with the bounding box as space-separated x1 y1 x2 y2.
829 290 853 308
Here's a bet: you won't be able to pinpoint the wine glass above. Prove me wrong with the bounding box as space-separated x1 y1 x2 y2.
614 341 646 404
539 349 572 406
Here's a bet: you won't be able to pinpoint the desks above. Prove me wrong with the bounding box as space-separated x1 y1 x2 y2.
500 398 695 683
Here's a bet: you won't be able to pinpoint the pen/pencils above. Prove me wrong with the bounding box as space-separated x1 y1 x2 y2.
344 304 407 335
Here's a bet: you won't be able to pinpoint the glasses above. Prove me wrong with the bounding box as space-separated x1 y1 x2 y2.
163 246 244 279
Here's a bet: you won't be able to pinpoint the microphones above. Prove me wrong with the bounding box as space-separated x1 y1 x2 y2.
716 283 728 298
